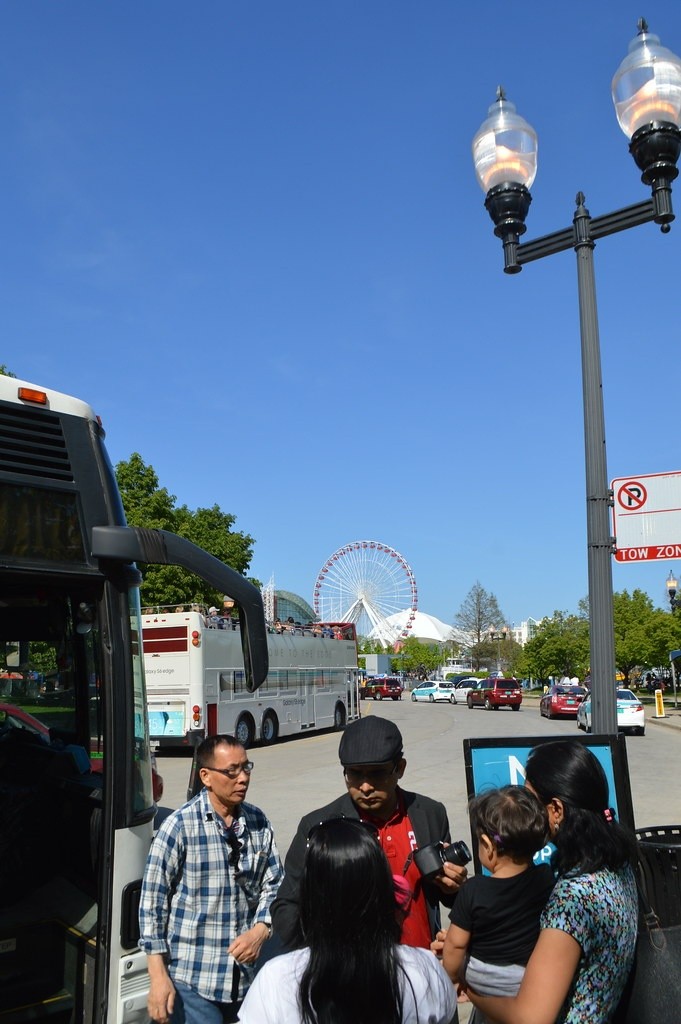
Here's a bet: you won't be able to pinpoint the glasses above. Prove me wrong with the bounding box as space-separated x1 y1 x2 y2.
343 760 398 785
204 761 254 778
305 818 381 852
223 828 240 867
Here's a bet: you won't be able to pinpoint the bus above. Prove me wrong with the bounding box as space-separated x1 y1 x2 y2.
0 368 271 1024
359 667 366 692
120 601 361 756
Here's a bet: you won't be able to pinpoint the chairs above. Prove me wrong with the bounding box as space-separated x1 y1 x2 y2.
206 615 241 631
294 628 304 636
303 630 313 637
283 629 293 636
314 631 323 638
323 631 330 638
621 694 629 699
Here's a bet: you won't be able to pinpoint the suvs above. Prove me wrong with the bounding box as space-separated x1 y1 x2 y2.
466 678 523 711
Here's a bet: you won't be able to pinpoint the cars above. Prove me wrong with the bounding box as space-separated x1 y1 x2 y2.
576 688 644 734
448 675 474 686
363 677 403 701
0 701 165 805
410 680 454 703
539 684 589 720
450 678 486 705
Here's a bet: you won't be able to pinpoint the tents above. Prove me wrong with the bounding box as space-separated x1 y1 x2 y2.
669 649 681 707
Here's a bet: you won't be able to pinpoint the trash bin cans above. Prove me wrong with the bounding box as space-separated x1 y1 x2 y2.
634 825 681 926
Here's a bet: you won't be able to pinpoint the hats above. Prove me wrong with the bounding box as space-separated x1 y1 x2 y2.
338 715 403 765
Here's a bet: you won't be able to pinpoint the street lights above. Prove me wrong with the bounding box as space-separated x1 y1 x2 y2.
467 11 681 735
489 627 508 677
666 569 679 617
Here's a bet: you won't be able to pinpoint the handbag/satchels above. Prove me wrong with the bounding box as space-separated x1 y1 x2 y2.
623 852 680 1024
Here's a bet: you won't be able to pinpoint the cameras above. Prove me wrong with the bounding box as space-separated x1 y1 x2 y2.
412 841 471 882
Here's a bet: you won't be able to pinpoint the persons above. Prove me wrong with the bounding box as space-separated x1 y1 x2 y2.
513 675 516 680
190 606 205 621
660 680 665 694
163 608 168 613
176 606 184 612
430 740 638 1024
441 784 557 998
264 617 340 638
223 610 237 631
237 819 458 1024
209 606 224 629
523 678 527 689
646 673 653 694
137 734 286 1024
270 716 468 949
146 608 153 615
